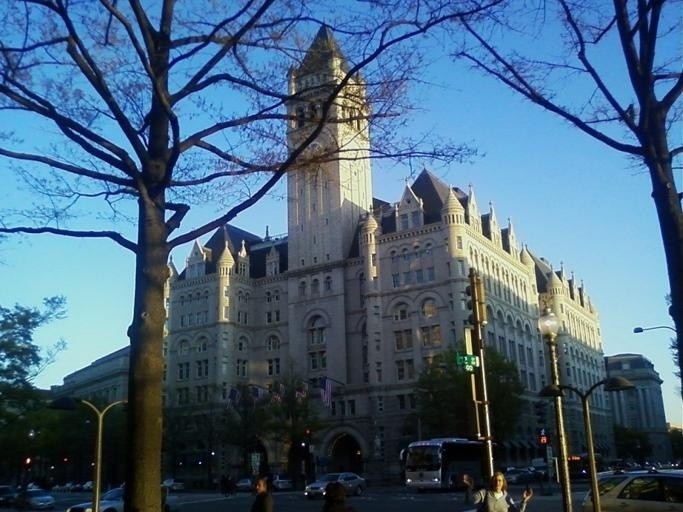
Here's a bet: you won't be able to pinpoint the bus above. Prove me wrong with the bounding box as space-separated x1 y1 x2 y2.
400 438 497 494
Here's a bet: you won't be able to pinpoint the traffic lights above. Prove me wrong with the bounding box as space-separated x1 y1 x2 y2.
23 455 32 465
303 428 312 434
466 276 488 325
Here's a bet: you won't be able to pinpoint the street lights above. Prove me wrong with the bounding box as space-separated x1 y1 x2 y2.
28 429 46 481
356 449 362 475
537 305 636 511
55 396 129 512
634 325 677 334
301 439 316 484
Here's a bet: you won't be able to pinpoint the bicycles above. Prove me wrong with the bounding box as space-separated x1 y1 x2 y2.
225 484 234 497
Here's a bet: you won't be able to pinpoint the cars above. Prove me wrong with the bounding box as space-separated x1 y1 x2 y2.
235 474 294 492
582 461 683 512
162 478 184 491
503 463 552 486
304 472 367 499
0 481 125 512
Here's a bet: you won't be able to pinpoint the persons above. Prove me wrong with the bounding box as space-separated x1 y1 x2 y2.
462 471 533 512
250 477 275 512
322 482 358 512
